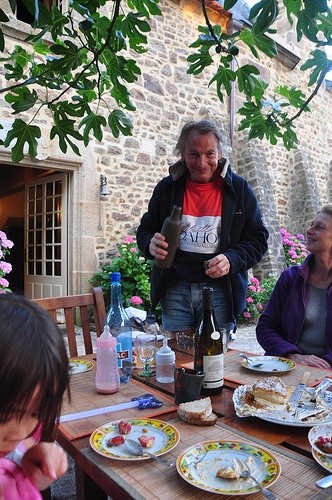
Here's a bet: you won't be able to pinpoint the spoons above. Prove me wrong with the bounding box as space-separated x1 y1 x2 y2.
123 438 167 463
231 455 279 500
237 353 263 368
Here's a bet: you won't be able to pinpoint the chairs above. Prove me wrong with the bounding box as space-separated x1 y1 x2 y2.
29 285 107 358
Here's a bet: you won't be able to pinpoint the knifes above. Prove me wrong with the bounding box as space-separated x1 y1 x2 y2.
286 371 311 403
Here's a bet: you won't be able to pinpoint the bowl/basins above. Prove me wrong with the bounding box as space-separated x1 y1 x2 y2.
307 424 332 457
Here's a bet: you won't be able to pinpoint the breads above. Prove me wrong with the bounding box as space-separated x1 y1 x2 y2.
178 397 218 425
246 375 288 408
216 466 239 479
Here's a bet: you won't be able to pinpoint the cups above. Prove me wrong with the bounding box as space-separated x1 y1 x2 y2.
219 328 228 354
174 331 191 348
174 367 208 405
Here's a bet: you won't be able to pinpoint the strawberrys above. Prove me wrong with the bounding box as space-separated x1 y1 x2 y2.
138 435 155 447
108 436 125 446
118 421 131 434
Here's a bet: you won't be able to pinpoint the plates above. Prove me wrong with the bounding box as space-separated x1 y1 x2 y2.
175 439 282 496
240 356 298 373
89 418 181 459
68 358 93 374
232 384 332 427
310 447 332 475
117 329 171 340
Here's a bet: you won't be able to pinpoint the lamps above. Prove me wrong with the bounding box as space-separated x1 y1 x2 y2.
97 174 111 197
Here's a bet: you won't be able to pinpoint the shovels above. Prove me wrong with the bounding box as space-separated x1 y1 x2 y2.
293 377 332 419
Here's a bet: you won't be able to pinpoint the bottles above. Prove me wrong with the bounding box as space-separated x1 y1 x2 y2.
153 207 183 270
105 272 133 383
155 338 176 384
95 325 121 395
193 288 225 397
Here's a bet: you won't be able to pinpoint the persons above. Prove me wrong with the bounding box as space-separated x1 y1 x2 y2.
0 293 72 500
255 203 332 371
136 119 270 347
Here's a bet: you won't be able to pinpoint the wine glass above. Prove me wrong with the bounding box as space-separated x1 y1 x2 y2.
136 333 157 376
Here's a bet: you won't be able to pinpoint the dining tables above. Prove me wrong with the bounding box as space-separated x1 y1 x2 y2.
52 338 332 500
